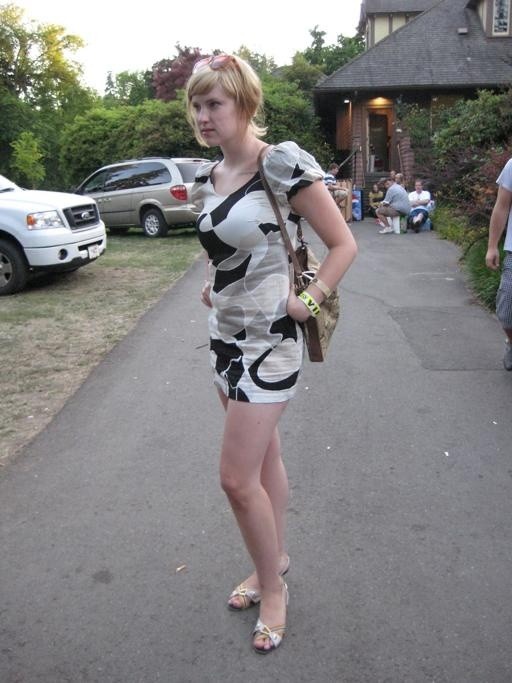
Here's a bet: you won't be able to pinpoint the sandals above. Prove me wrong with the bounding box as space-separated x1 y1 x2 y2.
228 555 290 655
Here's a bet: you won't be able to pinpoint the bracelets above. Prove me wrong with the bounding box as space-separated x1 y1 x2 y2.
298 291 321 318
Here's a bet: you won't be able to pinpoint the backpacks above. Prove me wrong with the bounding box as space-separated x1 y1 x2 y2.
293 246 340 363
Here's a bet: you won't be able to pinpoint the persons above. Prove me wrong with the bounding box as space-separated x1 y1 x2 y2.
324 163 349 208
486 158 512 370
186 53 358 654
368 170 431 234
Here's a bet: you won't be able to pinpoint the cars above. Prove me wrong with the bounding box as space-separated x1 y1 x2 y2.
0 175 106 296
71 157 210 238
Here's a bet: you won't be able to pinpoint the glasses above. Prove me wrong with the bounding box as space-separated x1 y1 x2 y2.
192 55 243 79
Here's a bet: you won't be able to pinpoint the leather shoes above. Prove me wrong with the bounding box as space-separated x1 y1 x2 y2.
414 220 419 233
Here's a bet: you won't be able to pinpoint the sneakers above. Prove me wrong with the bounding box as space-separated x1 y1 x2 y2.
503 345 512 370
379 227 392 234
